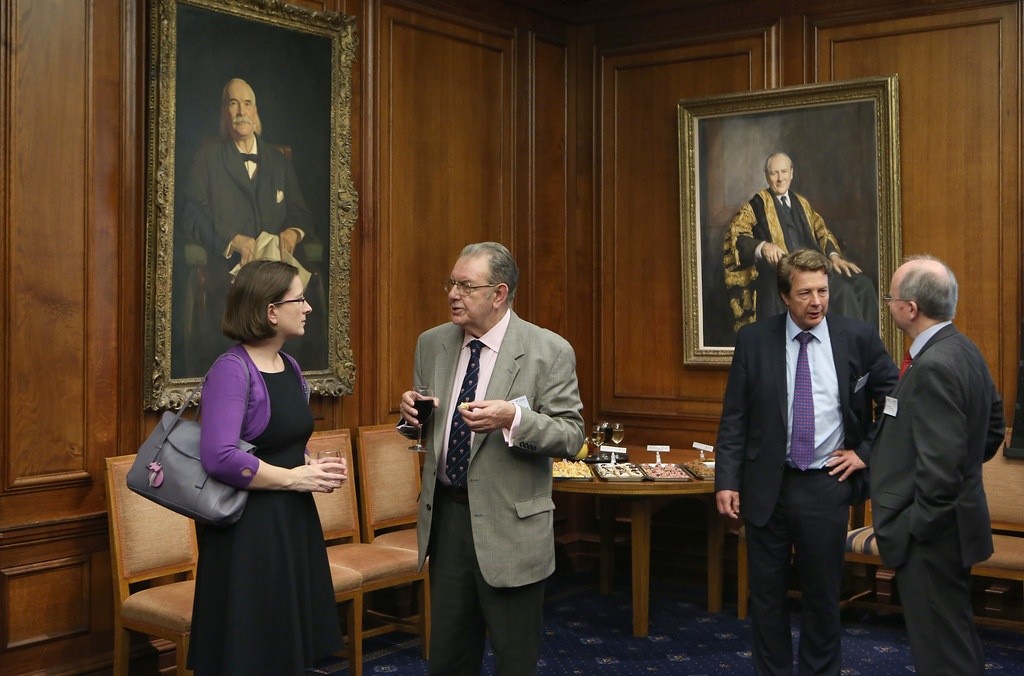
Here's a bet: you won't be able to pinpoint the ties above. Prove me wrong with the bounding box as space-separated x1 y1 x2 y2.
898 350 913 381
238 152 259 163
445 340 485 485
790 332 816 473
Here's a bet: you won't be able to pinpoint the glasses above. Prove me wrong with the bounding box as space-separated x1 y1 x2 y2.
271 295 305 307
881 293 919 307
443 280 510 297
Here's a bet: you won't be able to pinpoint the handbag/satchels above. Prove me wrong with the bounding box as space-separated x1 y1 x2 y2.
126 353 253 524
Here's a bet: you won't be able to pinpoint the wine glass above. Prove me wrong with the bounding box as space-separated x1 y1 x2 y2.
408 387 434 453
591 421 625 461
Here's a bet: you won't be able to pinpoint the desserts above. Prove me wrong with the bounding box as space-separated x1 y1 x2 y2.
553 458 716 479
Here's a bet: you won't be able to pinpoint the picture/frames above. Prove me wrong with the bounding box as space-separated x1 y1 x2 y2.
142 0 359 413
677 73 905 372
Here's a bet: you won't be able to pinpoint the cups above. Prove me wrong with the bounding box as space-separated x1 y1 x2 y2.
317 449 344 488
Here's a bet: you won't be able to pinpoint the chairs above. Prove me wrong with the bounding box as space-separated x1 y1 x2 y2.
305 423 488 676
843 457 1024 635
104 455 199 676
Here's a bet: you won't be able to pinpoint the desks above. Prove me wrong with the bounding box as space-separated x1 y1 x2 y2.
548 440 725 638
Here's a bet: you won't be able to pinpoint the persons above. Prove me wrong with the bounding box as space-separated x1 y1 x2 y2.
713 250 900 676
396 241 584 676
185 261 348 676
867 257 1007 676
